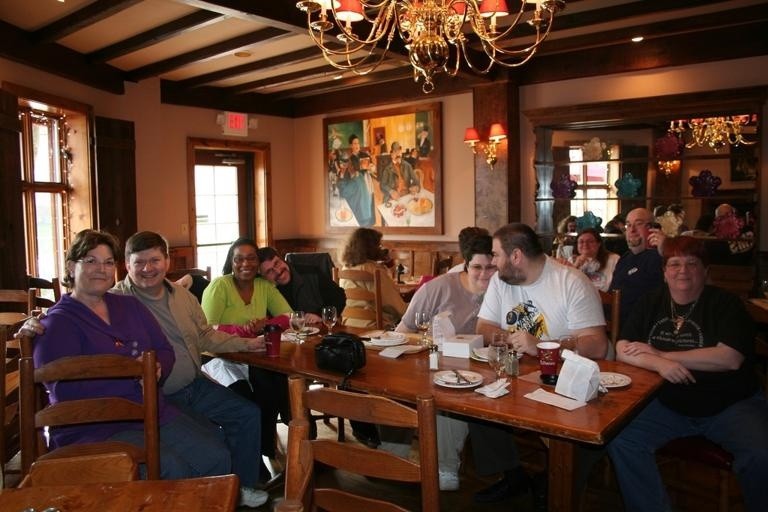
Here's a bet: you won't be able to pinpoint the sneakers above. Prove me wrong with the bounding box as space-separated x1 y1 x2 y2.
438 472 459 492
240 486 268 508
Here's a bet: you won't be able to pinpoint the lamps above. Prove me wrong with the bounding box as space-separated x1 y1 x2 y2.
296 0 567 94
667 114 760 153
464 124 508 171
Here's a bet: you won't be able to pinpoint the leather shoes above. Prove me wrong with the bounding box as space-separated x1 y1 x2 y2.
533 474 548 509
473 477 528 503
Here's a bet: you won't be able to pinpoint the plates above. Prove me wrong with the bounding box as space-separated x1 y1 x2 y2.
407 197 434 216
433 370 482 388
370 331 410 347
286 327 320 336
599 371 631 387
335 207 353 222
470 353 523 363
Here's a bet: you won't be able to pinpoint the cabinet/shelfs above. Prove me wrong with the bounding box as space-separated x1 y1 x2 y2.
555 116 751 265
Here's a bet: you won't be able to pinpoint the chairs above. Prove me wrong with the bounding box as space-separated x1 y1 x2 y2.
0 249 768 512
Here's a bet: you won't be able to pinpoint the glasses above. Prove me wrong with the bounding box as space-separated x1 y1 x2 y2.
378 243 383 248
668 261 697 271
233 256 257 264
75 256 115 270
624 222 644 231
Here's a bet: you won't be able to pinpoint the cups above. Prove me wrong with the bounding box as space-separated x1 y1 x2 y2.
492 333 508 368
264 324 281 358
644 221 662 251
411 276 423 284
558 334 577 364
536 341 561 377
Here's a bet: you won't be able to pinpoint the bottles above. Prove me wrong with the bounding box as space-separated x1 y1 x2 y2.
505 350 520 375
428 344 439 369
397 264 405 284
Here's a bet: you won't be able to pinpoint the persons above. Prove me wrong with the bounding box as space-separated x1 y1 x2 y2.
338 228 409 329
608 236 766 509
177 247 347 323
34 229 231 482
14 232 268 507
378 236 499 490
473 224 609 511
201 238 317 458
329 126 431 227
553 206 757 317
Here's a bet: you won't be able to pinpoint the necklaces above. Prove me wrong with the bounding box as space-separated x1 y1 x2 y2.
670 297 699 335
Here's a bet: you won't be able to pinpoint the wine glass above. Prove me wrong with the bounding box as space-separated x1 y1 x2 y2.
415 312 433 340
321 306 338 336
487 343 508 389
289 311 305 344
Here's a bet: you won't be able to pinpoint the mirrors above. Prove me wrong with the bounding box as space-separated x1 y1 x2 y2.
522 85 768 300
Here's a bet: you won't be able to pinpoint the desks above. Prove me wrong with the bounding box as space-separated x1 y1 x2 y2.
219 322 666 508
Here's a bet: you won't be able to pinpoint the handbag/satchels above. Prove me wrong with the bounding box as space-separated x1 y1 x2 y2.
315 332 368 372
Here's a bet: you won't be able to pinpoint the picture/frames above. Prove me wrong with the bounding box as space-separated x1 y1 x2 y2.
323 102 444 235
